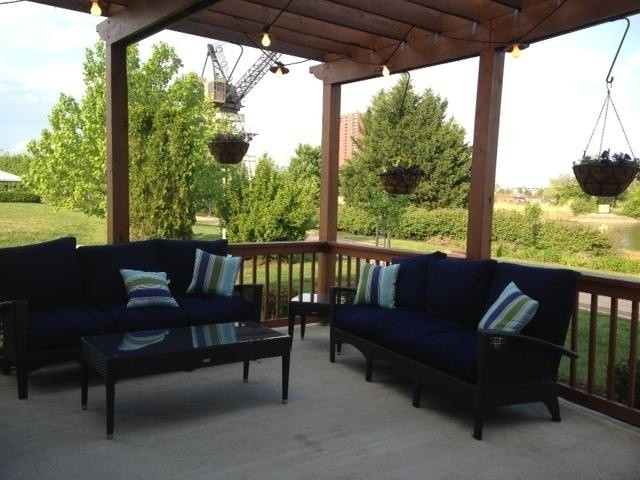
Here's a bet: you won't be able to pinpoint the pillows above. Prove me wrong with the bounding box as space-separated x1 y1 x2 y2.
118 329 170 350
120 267 183 308
189 323 239 349
185 247 242 299
477 281 541 350
351 261 403 310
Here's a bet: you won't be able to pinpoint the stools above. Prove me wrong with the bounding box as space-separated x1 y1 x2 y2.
287 292 355 353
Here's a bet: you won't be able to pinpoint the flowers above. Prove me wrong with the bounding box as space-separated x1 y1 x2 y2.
573 148 640 181
207 131 256 140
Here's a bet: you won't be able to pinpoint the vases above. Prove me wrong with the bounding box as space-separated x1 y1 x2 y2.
207 141 249 164
573 166 636 197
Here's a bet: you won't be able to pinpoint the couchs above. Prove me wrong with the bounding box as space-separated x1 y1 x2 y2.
0 235 265 401
328 250 583 440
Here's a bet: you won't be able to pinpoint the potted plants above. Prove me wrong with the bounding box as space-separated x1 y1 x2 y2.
380 157 429 194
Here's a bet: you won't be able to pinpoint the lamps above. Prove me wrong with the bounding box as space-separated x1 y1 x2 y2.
270 64 290 78
261 26 271 46
89 0 103 17
